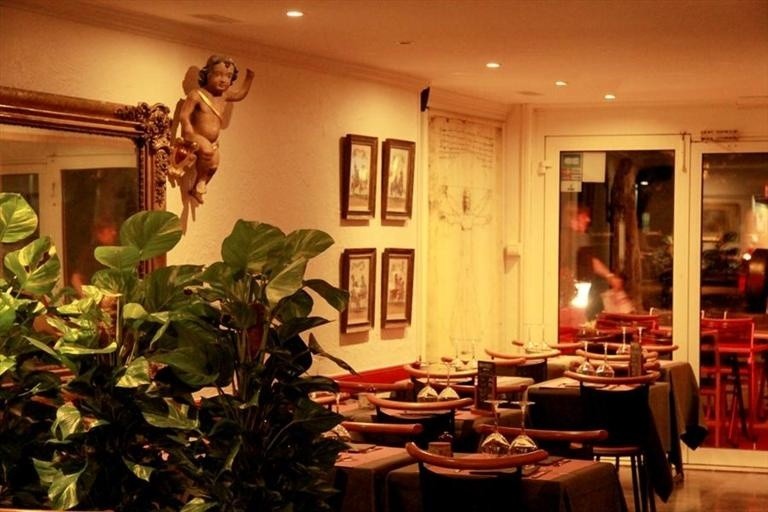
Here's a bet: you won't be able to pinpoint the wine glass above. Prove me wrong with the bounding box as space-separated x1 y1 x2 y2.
575 325 649 380
416 324 476 400
508 400 538 469
480 398 509 461
327 390 351 452
521 322 553 356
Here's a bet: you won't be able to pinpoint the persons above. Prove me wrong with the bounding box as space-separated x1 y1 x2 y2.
565 201 624 306
72 206 125 298
181 52 255 204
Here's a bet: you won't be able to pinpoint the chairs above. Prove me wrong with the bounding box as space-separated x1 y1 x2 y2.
312 307 768 512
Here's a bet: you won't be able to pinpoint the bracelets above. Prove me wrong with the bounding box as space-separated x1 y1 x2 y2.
605 273 615 280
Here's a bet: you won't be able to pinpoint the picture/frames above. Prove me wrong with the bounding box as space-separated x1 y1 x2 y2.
381 247 415 329
344 133 378 220
342 248 377 334
383 138 416 221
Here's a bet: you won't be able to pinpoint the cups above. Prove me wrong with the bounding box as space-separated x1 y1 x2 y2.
357 391 377 411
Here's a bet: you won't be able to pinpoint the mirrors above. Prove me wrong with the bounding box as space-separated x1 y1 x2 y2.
1 85 173 387
